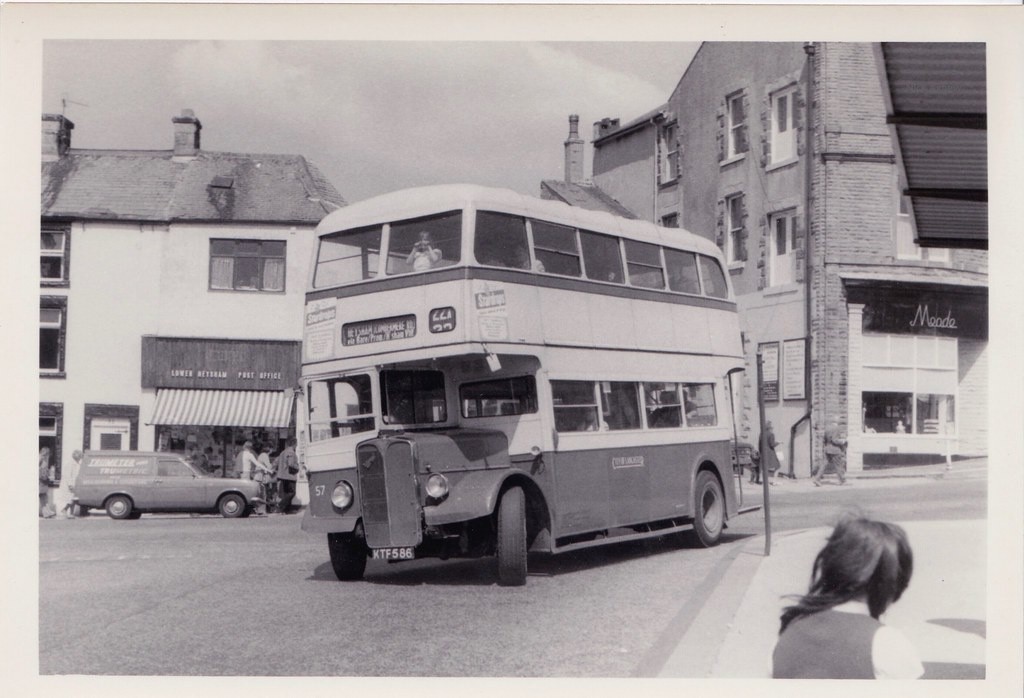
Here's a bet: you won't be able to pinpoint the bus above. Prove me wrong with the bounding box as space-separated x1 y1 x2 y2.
289 181 747 589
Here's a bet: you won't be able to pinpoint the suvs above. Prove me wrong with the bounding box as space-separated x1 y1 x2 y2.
70 449 264 520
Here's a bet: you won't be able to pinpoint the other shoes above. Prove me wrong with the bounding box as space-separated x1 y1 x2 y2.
68 514 75 518
840 481 849 485
61 509 67 517
813 480 821 487
770 482 778 486
275 503 281 512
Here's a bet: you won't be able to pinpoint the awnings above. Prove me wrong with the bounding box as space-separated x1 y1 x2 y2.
145 389 296 428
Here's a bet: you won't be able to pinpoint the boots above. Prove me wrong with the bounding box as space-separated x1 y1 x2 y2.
41 505 56 518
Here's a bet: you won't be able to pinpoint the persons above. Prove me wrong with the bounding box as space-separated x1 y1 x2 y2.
648 392 682 428
773 519 925 679
61 449 84 516
407 231 441 271
605 269 616 282
362 383 412 431
187 445 210 472
814 417 852 486
233 438 299 516
667 259 701 293
515 245 545 272
684 391 698 419
39 447 55 518
750 421 782 484
577 404 609 432
